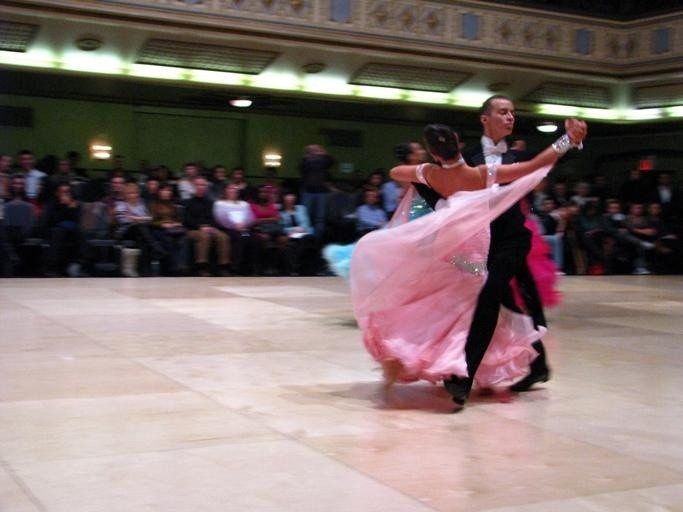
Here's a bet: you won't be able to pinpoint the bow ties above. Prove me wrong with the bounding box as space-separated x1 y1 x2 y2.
480 141 507 157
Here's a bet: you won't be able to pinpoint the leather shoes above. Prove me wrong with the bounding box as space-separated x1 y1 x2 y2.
442 371 471 406
510 360 551 393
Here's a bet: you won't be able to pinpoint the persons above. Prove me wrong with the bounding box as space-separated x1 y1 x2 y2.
346 117 589 403
411 93 590 403
